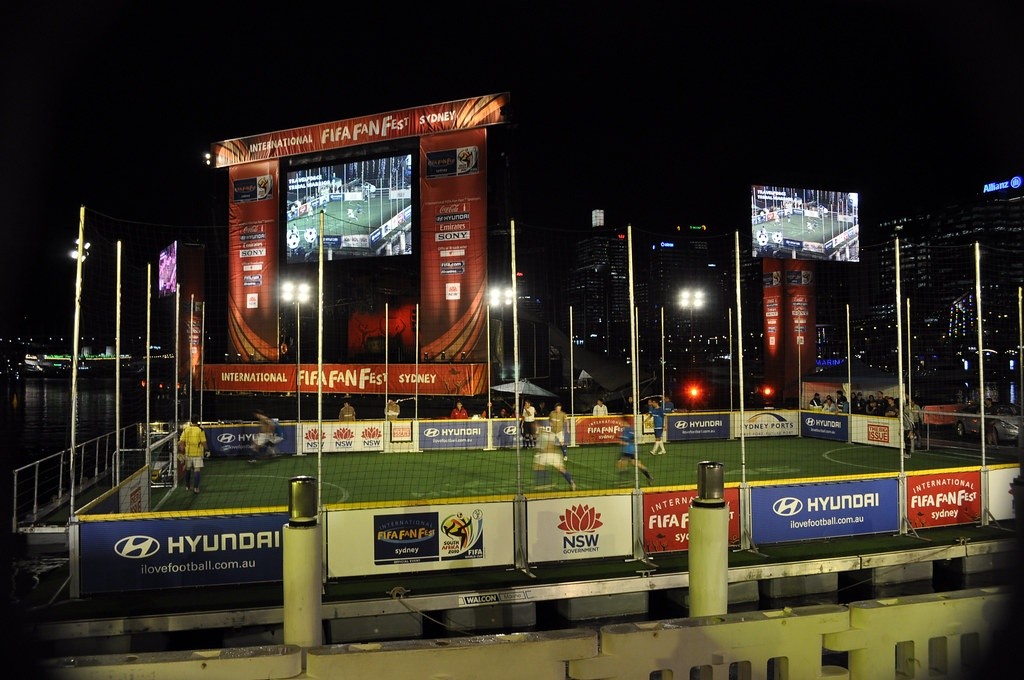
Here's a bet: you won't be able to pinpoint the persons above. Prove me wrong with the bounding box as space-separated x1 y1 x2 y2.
497 409 509 418
451 401 468 419
531 419 577 492
179 414 211 494
286 179 381 254
338 400 356 422
754 199 830 251
246 409 280 464
472 411 487 419
534 401 548 417
661 396 674 413
383 398 400 420
809 388 925 459
614 416 653 486
592 399 609 417
646 398 654 414
546 402 570 463
624 396 634 415
980 397 999 446
521 399 538 449
512 408 521 418
649 400 668 457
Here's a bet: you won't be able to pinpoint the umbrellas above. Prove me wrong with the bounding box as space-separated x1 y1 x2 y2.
489 378 560 408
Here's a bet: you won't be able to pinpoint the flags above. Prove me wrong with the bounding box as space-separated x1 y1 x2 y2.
157 242 178 296
